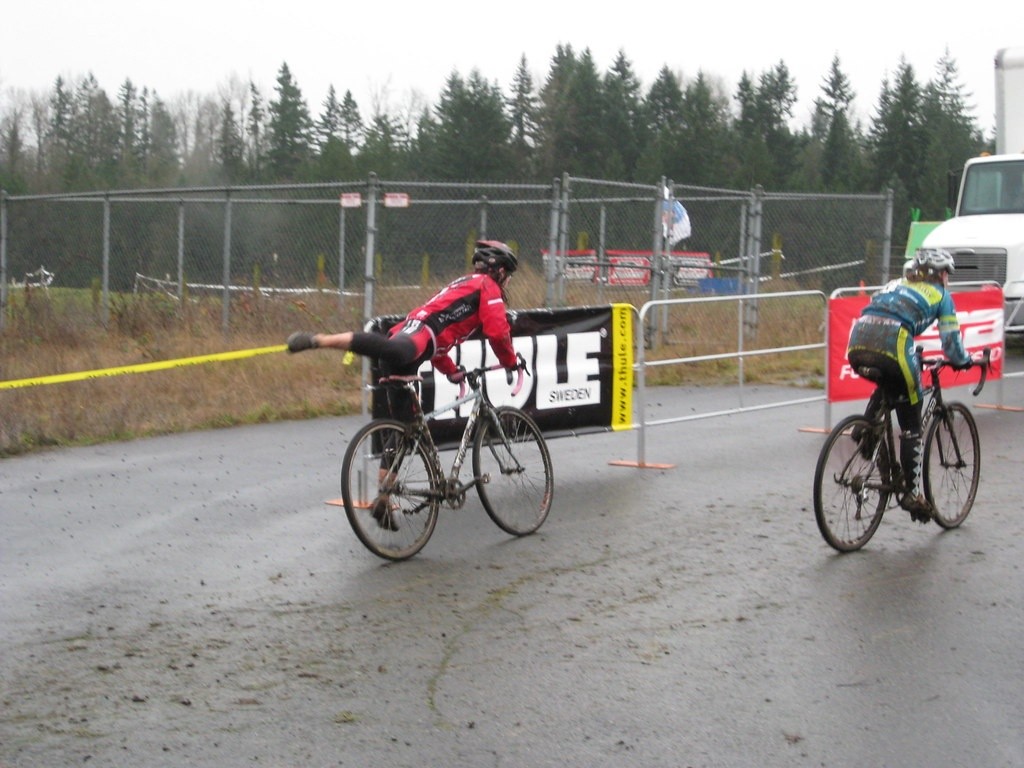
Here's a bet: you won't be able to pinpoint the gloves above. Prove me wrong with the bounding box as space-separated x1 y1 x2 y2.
957 358 975 371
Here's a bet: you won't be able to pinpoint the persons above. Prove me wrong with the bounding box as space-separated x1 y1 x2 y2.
847 249 973 517
287 240 518 530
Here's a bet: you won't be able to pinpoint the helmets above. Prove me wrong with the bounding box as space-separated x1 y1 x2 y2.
915 246 957 276
473 239 519 277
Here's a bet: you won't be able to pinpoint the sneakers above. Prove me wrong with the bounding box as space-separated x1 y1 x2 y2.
287 330 314 354
369 496 401 531
900 491 936 519
851 422 876 460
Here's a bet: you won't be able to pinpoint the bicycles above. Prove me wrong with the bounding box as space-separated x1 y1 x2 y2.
812 345 992 554
340 352 556 563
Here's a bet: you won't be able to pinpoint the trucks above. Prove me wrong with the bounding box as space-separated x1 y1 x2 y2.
902 149 1024 348
